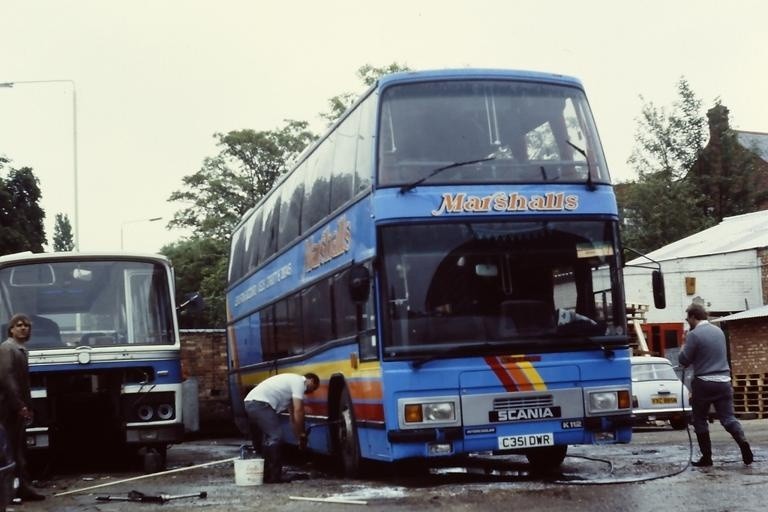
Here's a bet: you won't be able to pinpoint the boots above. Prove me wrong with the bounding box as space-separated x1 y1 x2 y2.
725 422 754 466
690 430 714 466
265 446 290 482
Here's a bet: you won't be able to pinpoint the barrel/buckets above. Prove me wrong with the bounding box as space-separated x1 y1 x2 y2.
233 459 264 486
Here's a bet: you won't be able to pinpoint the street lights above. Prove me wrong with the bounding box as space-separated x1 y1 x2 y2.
0 75 85 255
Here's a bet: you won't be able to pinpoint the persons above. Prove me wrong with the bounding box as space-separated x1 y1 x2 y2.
677 302 753 467
243 372 319 482
0 313 52 502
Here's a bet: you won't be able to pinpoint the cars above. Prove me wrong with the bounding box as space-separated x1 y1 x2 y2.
631 355 693 433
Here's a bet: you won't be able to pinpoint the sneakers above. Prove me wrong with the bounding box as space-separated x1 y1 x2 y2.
6 494 22 505
18 491 48 502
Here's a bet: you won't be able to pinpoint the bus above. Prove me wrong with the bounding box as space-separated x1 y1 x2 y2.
221 65 667 482
0 249 200 472
